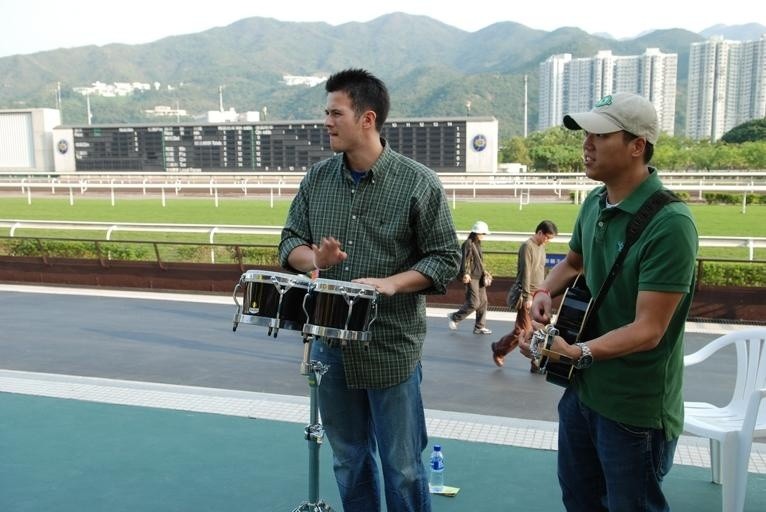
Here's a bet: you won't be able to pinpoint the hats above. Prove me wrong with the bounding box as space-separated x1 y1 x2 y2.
471 221 491 234
563 91 659 145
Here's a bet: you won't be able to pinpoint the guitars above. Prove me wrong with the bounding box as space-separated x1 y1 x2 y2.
528 273 594 388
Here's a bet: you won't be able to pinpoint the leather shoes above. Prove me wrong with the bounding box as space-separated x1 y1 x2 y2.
492 342 504 367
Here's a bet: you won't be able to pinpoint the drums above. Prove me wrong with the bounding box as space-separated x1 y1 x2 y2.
236 270 311 330
302 278 376 342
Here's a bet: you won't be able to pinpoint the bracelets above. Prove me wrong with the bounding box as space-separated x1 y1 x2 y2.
313 253 333 271
533 288 551 297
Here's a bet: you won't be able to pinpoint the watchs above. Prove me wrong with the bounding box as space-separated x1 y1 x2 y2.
574 343 594 370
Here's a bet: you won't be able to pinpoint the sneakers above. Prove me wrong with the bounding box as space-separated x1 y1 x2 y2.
447 313 457 330
473 326 493 334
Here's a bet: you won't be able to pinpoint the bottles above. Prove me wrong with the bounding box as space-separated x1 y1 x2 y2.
428 443 444 492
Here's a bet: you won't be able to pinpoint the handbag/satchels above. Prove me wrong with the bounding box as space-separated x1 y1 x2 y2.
483 270 494 287
506 284 523 312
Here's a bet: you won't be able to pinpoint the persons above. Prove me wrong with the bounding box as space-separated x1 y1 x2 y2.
278 67 463 512
520 94 699 510
448 220 492 334
491 220 558 373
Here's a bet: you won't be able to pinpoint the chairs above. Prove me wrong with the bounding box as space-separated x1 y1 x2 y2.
679 328 766 512
